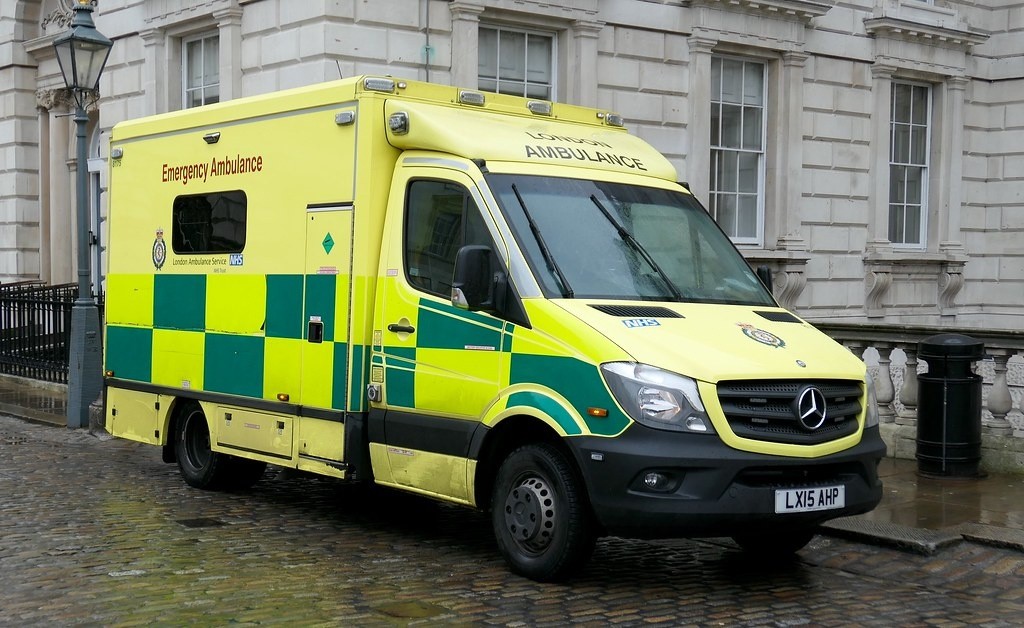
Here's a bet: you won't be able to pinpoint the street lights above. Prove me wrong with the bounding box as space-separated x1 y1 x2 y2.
53 4 114 429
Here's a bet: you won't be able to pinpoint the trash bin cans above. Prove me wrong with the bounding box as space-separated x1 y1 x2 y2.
914 332 990 480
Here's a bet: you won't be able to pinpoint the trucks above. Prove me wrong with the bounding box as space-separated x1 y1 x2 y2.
103 74 887 582
